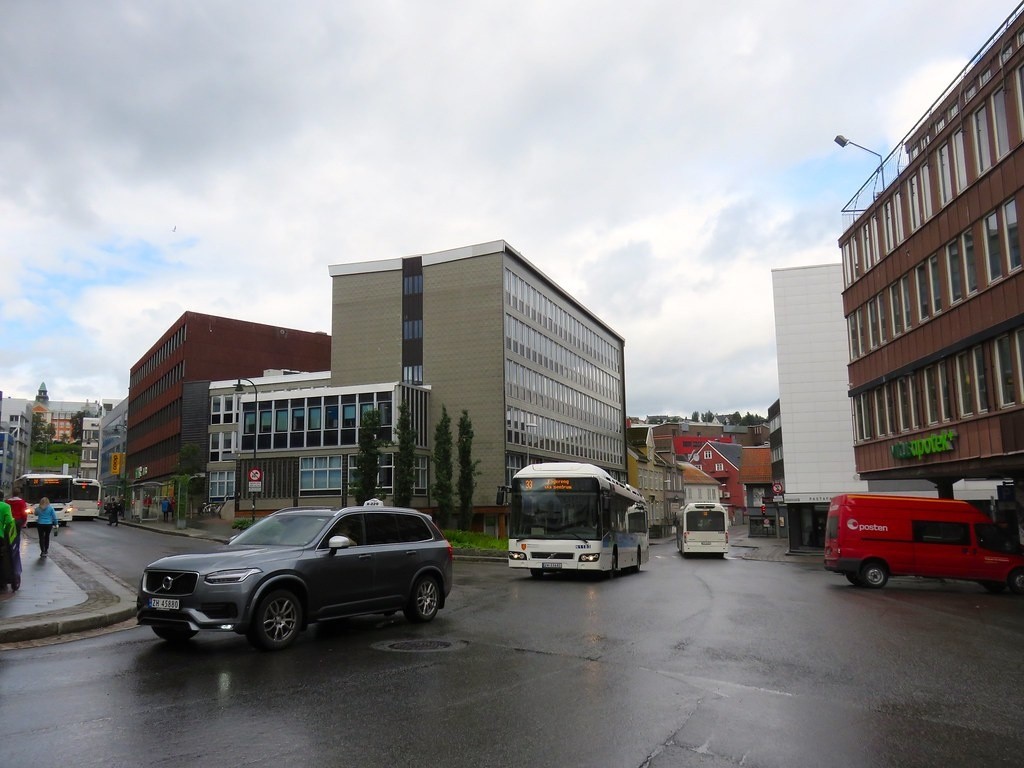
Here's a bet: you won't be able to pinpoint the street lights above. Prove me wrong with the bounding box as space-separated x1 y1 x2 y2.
834 137 885 192
113 424 128 519
232 376 259 520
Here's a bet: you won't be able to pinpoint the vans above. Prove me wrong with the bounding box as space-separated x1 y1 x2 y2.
825 494 1024 595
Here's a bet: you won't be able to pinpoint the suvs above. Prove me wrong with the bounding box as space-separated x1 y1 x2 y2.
135 499 454 652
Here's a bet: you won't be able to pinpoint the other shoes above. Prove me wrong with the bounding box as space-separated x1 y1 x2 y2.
114 524 118 527
107 523 112 526
40 552 48 556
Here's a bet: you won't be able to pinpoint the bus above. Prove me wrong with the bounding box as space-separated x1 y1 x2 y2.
495 462 650 577
11 474 73 526
72 478 101 521
674 503 729 558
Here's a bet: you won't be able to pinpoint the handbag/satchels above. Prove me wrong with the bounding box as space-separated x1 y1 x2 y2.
167 504 173 512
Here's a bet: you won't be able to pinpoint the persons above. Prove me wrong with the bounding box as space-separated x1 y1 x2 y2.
161 496 170 522
143 494 152 514
119 499 122 514
338 521 358 545
5 490 27 537
0 489 13 594
34 497 58 556
170 497 176 518
106 498 118 527
